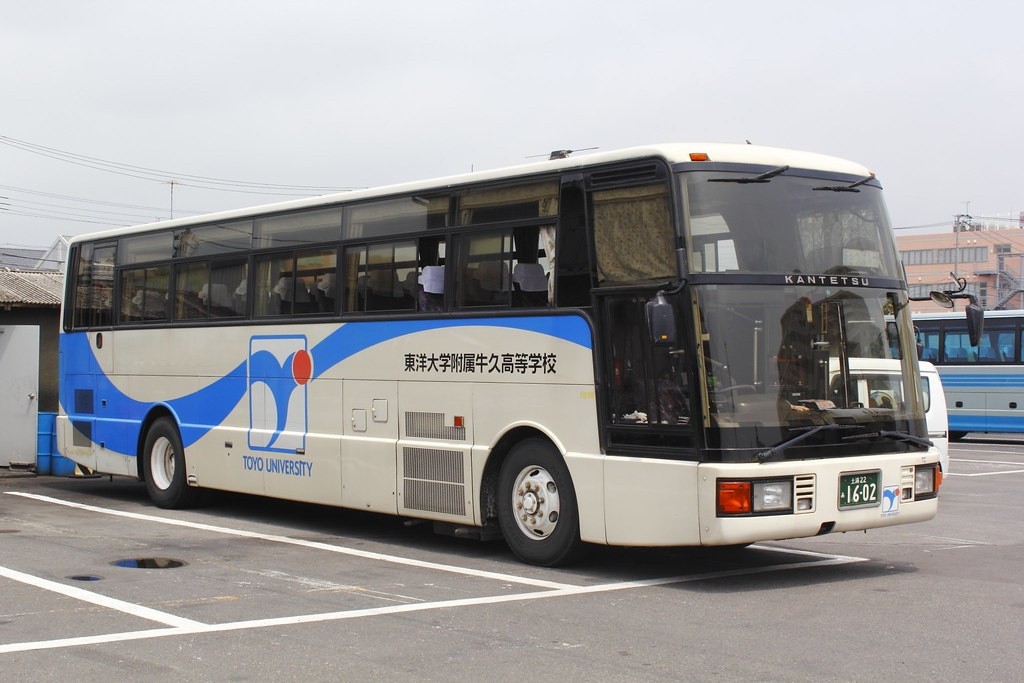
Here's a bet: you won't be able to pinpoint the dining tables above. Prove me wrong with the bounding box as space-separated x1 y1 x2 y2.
198 284 233 317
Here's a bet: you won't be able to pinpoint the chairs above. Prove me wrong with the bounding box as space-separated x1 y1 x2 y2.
368 270 408 310
973 346 996 361
922 349 930 363
929 348 938 362
945 347 958 362
272 278 310 314
467 268 479 306
513 263 549 308
956 348 968 362
1003 345 1014 362
424 265 444 312
316 274 335 312
358 276 367 311
479 261 510 305
646 301 757 424
166 290 199 320
234 279 248 315
407 272 425 310
132 289 164 321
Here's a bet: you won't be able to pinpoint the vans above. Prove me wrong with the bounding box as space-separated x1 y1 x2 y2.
828 356 951 481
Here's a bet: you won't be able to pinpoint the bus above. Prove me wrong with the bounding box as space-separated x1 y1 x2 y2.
883 308 1024 441
53 141 986 570
